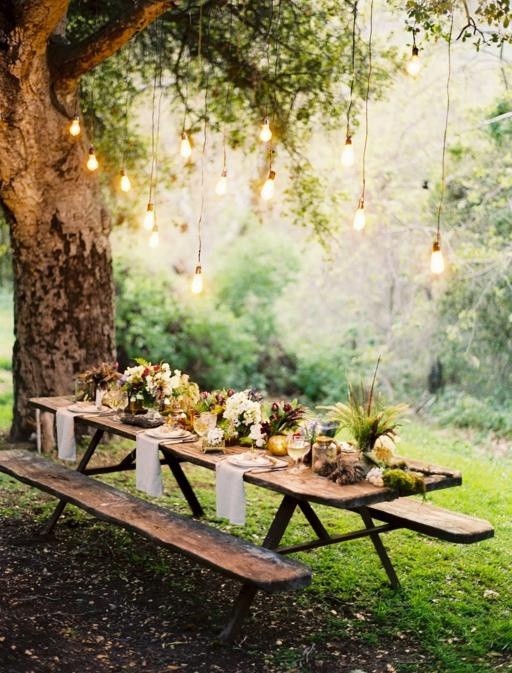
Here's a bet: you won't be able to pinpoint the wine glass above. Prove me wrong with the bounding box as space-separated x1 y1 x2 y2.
193 411 218 449
286 435 311 475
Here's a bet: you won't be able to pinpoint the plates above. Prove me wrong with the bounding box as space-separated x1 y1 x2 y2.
145 427 190 439
67 403 111 414
227 453 277 468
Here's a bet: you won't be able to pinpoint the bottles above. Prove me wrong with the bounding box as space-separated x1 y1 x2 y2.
311 436 337 476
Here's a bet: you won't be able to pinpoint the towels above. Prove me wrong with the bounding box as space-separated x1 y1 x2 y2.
216 450 288 526
55 403 113 462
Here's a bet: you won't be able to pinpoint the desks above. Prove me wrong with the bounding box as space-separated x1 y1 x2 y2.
26 394 466 646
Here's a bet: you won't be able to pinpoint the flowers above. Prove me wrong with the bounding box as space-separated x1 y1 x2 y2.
72 355 434 501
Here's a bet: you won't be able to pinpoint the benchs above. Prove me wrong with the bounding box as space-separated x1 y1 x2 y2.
346 495 495 543
0 447 314 595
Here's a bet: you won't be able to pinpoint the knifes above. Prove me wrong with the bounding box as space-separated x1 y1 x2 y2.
251 468 288 473
